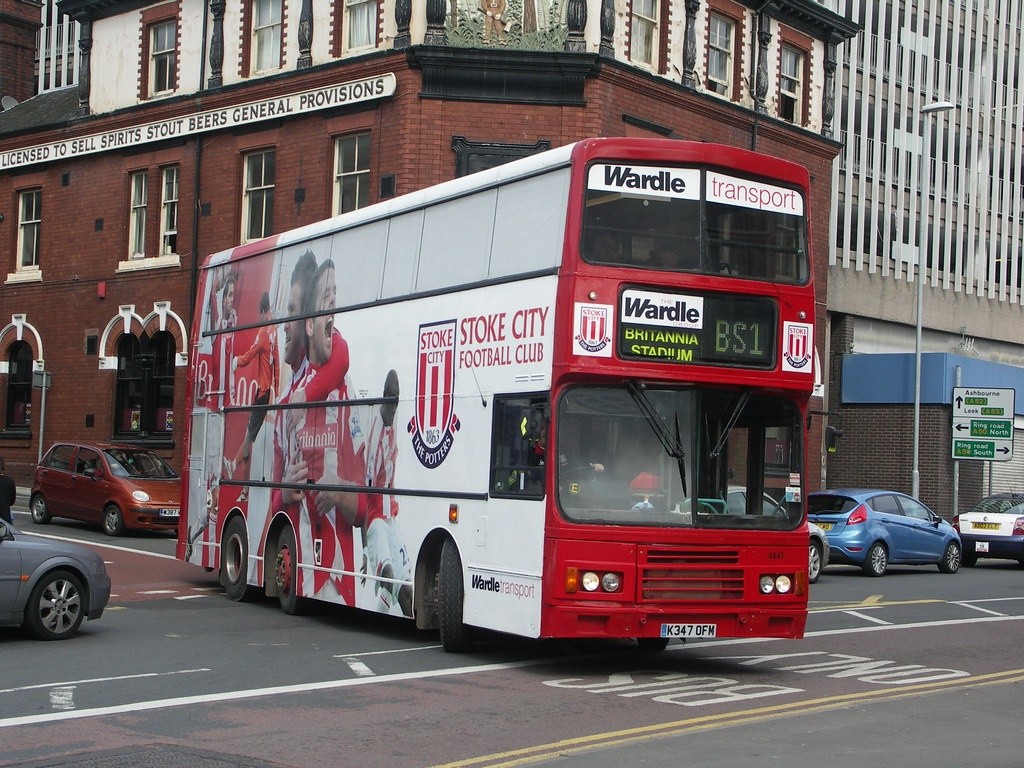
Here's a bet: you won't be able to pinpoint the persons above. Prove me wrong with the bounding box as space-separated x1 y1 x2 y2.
509 410 604 490
222 292 280 502
199 278 235 514
358 369 414 612
270 253 365 601
0 456 16 526
586 232 618 263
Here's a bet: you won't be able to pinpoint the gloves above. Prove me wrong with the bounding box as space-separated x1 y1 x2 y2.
232 356 239 372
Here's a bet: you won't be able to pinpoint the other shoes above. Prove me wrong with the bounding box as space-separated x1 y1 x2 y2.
223 457 234 480
236 489 248 502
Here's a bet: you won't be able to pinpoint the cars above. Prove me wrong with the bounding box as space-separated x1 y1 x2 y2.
808 489 962 584
29 441 181 535
952 493 1024 568
726 485 830 584
0 518 110 641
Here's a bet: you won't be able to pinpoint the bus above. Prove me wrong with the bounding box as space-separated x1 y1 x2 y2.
175 138 844 655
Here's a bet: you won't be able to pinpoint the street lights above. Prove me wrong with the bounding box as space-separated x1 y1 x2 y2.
912 102 954 500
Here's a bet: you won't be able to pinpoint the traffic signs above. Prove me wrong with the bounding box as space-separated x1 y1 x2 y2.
951 387 1016 461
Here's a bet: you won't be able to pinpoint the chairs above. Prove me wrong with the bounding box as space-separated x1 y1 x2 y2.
87 459 97 469
